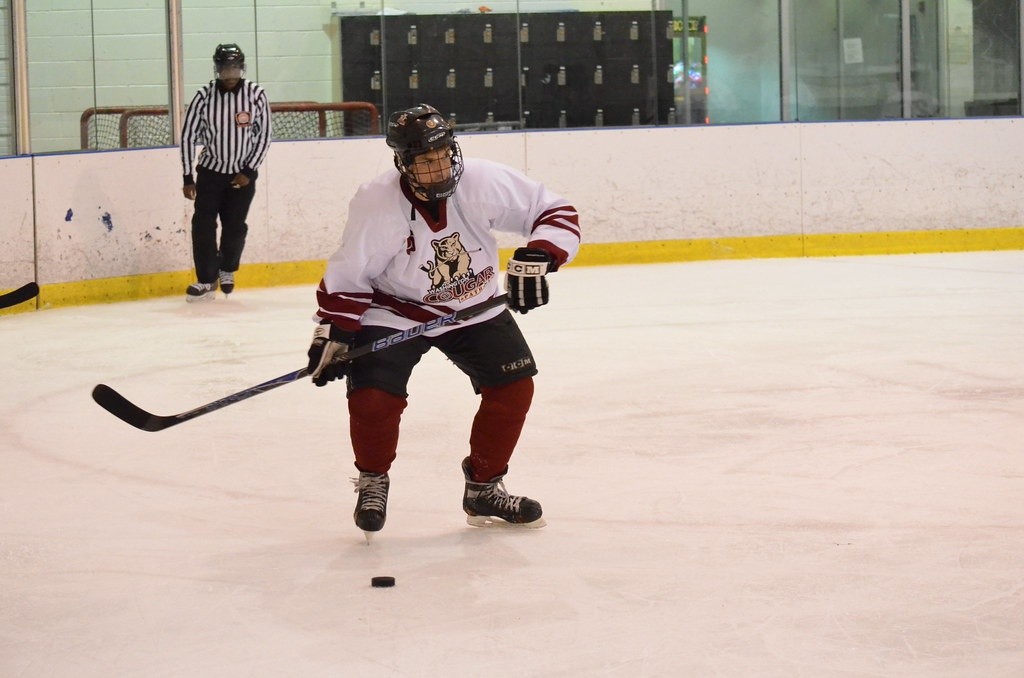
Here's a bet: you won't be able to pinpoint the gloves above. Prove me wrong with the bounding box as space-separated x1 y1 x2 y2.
504 247 551 315
308 323 353 386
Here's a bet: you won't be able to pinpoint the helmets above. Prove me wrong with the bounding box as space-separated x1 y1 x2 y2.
212 43 245 66
384 103 464 200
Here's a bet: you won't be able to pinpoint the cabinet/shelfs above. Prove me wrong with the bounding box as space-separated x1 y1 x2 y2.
332 9 677 135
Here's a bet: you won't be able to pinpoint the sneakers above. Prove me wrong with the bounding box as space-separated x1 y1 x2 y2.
461 456 548 529
219 270 235 298
186 280 218 302
349 462 390 544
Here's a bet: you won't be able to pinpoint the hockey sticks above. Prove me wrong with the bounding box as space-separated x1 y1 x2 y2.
91 291 509 432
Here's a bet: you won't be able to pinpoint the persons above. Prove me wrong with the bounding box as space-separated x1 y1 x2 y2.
180 42 272 303
307 103 581 546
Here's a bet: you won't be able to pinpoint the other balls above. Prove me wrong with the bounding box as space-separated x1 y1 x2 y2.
371 574 396 589
